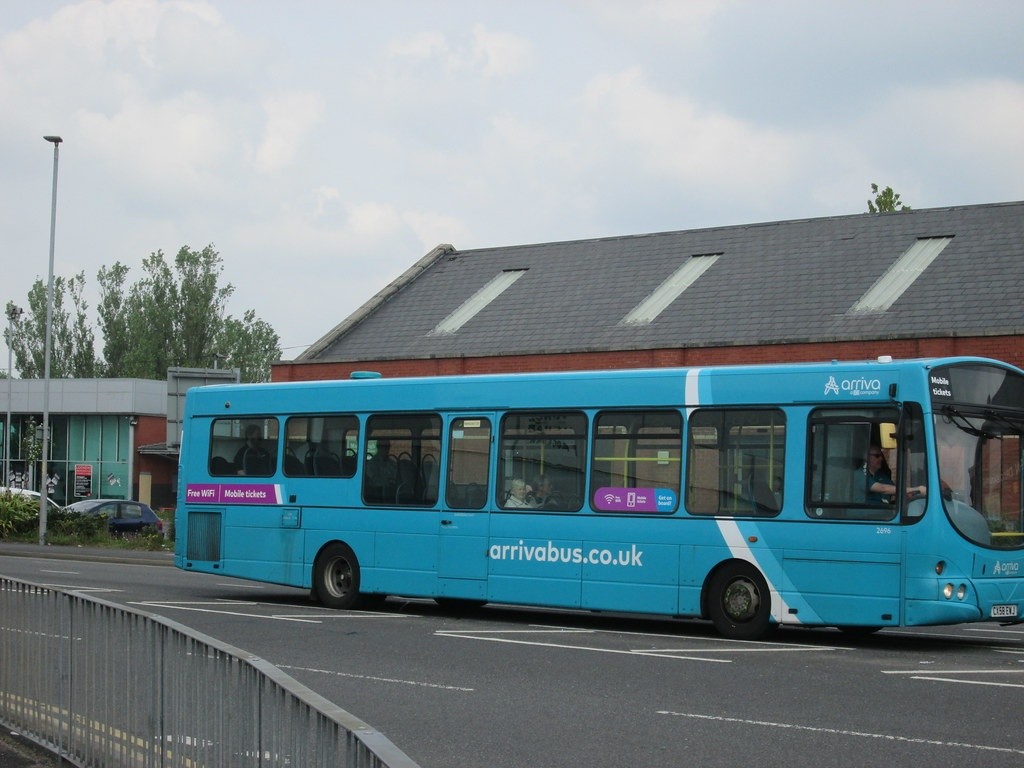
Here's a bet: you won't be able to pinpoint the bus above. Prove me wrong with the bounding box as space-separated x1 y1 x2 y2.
170 352 1024 640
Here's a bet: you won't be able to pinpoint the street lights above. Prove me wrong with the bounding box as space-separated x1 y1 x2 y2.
38 133 64 544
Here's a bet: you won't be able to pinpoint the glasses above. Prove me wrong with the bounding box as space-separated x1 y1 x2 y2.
870 453 884 458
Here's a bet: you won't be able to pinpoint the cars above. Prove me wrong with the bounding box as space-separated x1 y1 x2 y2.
0 486 63 520
59 498 165 541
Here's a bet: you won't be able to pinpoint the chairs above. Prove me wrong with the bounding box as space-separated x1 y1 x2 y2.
212 443 581 514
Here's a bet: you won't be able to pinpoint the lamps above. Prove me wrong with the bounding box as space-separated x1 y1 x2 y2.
129 416 137 425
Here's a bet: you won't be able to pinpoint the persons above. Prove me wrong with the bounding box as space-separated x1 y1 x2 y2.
845 447 926 516
503 478 535 509
528 474 557 507
232 425 262 475
366 438 399 487
